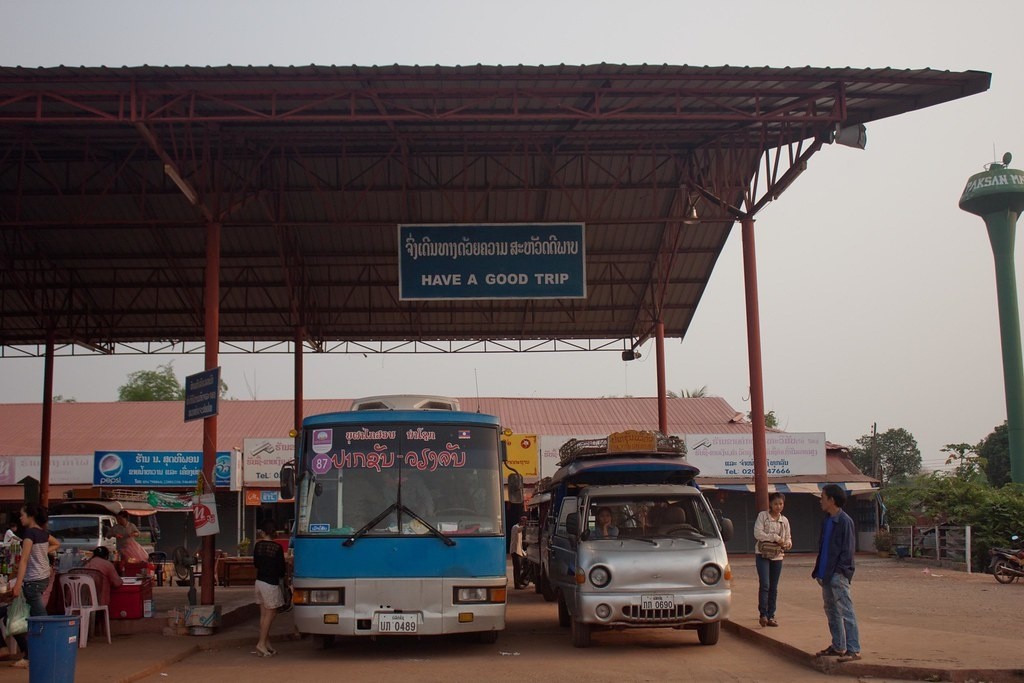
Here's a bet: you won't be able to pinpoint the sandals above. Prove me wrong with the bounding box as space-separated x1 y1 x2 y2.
816 645 848 656
837 650 861 662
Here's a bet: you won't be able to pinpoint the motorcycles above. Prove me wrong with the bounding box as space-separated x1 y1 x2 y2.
989 536 1024 584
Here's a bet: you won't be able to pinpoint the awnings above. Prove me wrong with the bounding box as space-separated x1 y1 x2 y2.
699 482 884 498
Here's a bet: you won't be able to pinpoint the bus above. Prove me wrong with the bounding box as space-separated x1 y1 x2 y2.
280 394 524 650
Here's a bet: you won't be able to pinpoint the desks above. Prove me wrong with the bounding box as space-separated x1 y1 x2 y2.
223 560 256 587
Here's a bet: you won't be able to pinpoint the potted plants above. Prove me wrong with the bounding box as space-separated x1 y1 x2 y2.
873 528 895 558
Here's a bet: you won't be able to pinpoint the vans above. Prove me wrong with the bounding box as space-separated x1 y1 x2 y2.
540 430 732 647
524 476 556 602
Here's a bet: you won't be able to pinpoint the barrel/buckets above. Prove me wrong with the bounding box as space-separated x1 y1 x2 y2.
25 614 82 683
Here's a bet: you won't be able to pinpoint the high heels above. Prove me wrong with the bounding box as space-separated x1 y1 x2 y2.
255 644 277 657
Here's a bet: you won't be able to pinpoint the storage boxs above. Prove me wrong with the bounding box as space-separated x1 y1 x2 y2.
185 604 222 627
143 599 157 617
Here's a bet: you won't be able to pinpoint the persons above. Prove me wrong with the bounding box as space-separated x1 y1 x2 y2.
66 546 123 641
812 485 862 662
4 522 23 543
253 520 286 657
754 493 792 628
510 516 530 590
3 502 61 666
106 511 141 561
589 508 619 540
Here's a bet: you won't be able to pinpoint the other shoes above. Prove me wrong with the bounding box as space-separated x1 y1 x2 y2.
8 658 30 669
759 617 778 627
515 586 524 590
521 576 530 580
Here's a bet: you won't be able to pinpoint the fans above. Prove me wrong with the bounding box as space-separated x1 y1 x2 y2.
171 546 198 605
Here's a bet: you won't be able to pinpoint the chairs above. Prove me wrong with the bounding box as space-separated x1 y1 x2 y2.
149 551 167 581
652 506 694 537
60 569 112 648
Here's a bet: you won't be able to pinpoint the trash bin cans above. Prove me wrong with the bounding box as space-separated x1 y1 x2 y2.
25 614 82 683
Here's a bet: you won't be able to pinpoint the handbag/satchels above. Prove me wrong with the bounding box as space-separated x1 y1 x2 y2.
277 577 293 614
6 590 30 636
757 542 783 559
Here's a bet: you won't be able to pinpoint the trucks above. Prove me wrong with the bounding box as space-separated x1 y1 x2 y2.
46 499 160 573
